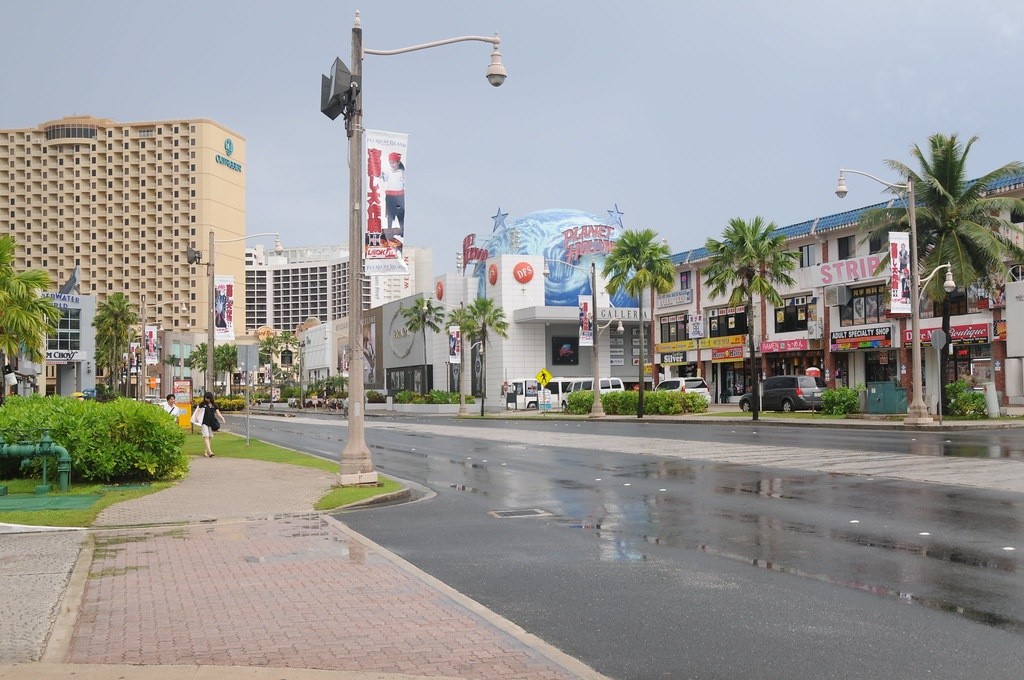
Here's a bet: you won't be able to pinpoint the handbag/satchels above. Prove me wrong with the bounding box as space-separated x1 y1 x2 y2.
211 418 220 431
190 406 205 426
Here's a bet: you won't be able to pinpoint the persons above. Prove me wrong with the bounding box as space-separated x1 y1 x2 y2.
323 396 333 411
291 398 296 409
195 392 226 458
161 393 180 424
335 399 343 412
381 152 406 237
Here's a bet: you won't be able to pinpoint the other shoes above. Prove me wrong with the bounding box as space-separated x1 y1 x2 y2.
204 453 209 457
209 452 215 457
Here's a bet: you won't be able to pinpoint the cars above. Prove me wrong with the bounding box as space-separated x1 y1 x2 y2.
654 376 712 409
557 375 626 414
737 374 831 414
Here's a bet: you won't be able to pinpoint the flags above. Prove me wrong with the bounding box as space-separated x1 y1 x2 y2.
214 274 235 340
887 231 911 314
364 129 409 278
578 294 593 347
121 324 158 374
449 325 462 364
264 364 272 384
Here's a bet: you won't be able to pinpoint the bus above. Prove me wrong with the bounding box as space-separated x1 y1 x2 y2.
507 375 574 412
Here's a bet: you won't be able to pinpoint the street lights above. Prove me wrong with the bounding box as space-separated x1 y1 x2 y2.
834 168 957 425
184 228 286 406
124 320 164 399
337 9 508 488
420 296 484 417
542 257 626 419
140 293 188 405
